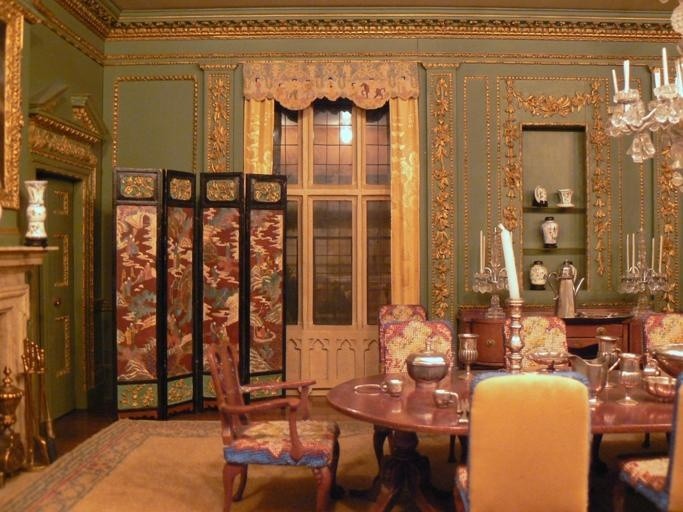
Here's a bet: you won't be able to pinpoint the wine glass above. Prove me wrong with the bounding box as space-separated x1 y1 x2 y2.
617 353 642 407
455 333 480 380
525 351 567 373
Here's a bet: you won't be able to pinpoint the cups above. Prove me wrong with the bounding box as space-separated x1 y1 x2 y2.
379 397 402 415
557 189 574 203
380 374 404 397
432 389 459 408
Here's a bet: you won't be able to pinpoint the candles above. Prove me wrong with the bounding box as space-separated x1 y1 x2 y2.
497 223 524 318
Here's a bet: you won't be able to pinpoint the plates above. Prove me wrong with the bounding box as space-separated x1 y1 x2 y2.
533 184 547 204
556 203 574 207
576 311 635 321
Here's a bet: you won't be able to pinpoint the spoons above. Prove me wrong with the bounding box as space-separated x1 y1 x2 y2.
353 381 379 393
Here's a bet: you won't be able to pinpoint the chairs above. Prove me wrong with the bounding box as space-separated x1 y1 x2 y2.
612 373 683 511
378 301 425 324
371 321 468 500
641 312 683 352
503 316 569 369
206 341 341 512
453 373 592 512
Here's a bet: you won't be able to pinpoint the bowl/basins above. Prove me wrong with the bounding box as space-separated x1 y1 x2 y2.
648 342 683 378
642 374 676 402
405 360 449 388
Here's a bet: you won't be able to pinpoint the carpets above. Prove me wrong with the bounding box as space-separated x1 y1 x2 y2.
0 417 621 512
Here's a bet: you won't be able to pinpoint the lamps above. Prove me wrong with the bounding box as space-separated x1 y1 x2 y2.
471 227 515 318
604 0 682 164
617 233 669 315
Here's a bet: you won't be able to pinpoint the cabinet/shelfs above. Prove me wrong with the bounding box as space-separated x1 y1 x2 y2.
521 206 589 290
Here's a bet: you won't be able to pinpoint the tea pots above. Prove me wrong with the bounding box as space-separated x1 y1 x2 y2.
567 348 621 408
642 351 661 377
545 257 585 318
594 335 621 365
